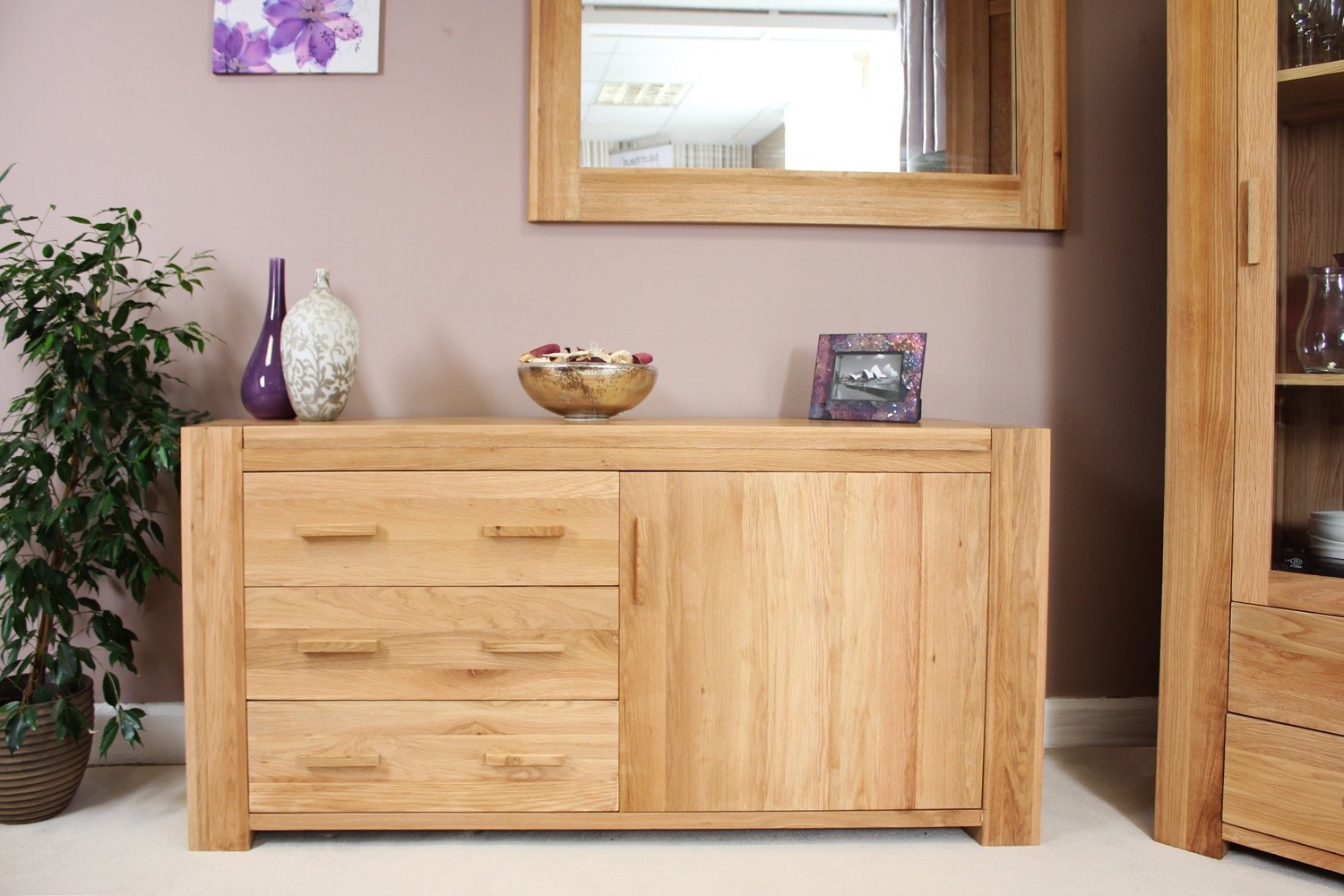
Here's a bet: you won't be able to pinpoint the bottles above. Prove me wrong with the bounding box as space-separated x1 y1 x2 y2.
240 258 296 420
279 267 357 422
1295 264 1344 373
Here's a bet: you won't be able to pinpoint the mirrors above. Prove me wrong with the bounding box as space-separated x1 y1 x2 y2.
528 0 1071 233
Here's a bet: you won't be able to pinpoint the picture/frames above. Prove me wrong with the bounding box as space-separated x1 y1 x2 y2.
809 333 927 424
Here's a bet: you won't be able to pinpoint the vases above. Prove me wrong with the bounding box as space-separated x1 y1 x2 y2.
280 268 359 423
241 258 298 421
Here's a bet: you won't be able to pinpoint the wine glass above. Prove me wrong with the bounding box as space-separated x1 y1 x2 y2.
1282 0 1344 67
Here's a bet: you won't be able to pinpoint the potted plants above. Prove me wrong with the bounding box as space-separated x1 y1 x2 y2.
0 164 227 825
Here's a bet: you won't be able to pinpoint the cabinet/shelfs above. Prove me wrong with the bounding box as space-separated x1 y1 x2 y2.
181 419 1052 850
1154 0 1344 875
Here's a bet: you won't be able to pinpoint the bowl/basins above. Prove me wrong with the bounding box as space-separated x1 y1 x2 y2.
518 362 658 422
1307 509 1344 568
